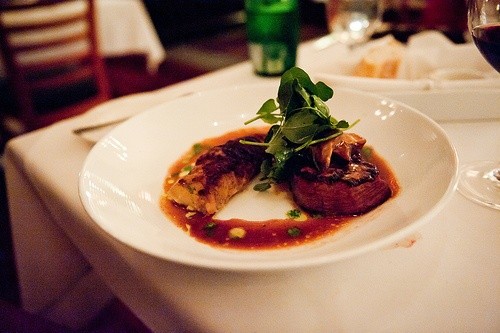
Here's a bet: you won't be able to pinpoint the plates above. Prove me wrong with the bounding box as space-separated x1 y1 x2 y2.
79 79 460 271
76 93 185 143
316 43 500 121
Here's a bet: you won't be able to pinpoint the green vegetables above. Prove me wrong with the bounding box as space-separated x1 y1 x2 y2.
235 65 361 190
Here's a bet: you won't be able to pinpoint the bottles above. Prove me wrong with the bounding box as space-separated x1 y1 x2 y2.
244 0 298 78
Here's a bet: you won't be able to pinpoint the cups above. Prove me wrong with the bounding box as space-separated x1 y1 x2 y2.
327 0 379 44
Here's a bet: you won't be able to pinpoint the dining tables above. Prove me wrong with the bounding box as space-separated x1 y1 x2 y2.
4 34 500 333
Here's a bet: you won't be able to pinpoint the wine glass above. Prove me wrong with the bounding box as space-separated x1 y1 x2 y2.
457 0 500 210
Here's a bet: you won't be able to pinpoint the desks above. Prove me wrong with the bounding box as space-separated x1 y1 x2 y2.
0 0 168 75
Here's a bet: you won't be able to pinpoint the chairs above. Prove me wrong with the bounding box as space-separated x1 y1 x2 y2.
0 0 112 136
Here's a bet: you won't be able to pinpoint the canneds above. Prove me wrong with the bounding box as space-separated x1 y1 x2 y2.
244 0 301 77
